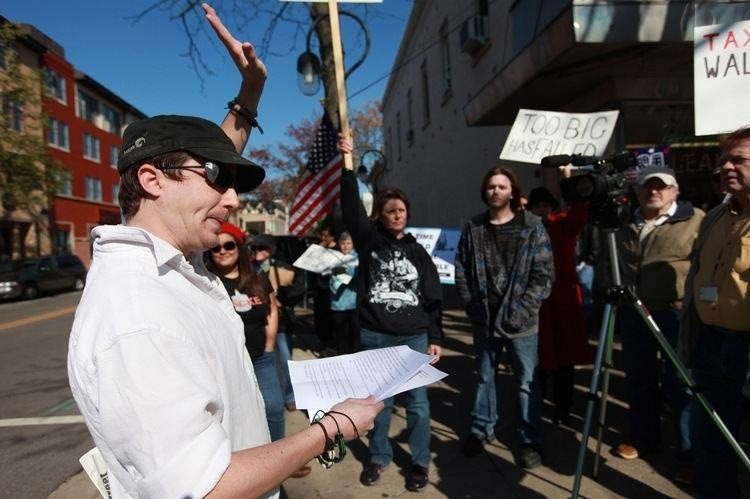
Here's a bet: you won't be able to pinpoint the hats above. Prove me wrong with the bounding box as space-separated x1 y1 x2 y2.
636 165 676 188
118 115 265 193
217 222 245 246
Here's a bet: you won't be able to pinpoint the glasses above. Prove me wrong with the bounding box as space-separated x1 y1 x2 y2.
211 241 238 254
155 160 239 197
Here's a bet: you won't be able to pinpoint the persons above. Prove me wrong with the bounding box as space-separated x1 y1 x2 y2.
616 165 706 459
523 186 594 440
697 163 726 213
678 127 750 499
452 166 557 471
206 218 358 478
334 129 445 492
65 2 386 498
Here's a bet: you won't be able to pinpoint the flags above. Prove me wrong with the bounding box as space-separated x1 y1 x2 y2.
286 108 346 242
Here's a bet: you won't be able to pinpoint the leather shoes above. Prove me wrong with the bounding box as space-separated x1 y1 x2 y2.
405 465 430 491
360 462 383 485
289 466 311 478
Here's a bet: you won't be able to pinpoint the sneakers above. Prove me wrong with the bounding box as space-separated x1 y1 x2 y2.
674 467 695 484
613 442 645 459
464 429 498 456
509 440 542 469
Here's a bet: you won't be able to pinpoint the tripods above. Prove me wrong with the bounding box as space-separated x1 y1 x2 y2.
567 233 749 498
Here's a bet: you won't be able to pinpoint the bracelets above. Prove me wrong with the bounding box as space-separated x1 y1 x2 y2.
224 95 264 135
309 409 360 470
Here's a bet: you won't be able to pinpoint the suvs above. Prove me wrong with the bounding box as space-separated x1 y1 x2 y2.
0 253 87 298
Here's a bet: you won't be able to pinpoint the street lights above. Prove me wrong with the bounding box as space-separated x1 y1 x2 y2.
289 11 370 209
359 148 387 199
